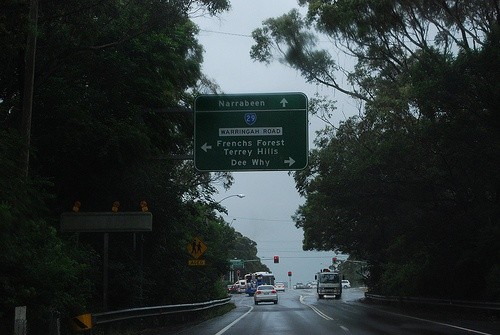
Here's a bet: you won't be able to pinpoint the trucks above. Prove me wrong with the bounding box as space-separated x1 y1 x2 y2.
314 271 342 299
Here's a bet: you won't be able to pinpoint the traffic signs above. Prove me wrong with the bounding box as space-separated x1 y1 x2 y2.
191 92 310 172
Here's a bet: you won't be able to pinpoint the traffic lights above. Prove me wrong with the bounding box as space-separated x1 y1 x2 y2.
274 256 280 263
332 258 337 264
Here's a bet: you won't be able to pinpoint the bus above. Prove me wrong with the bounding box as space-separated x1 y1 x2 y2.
245 272 276 296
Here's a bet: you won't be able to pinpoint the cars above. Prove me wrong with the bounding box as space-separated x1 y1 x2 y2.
294 282 314 288
234 280 246 289
341 280 352 288
254 285 278 305
275 283 285 291
